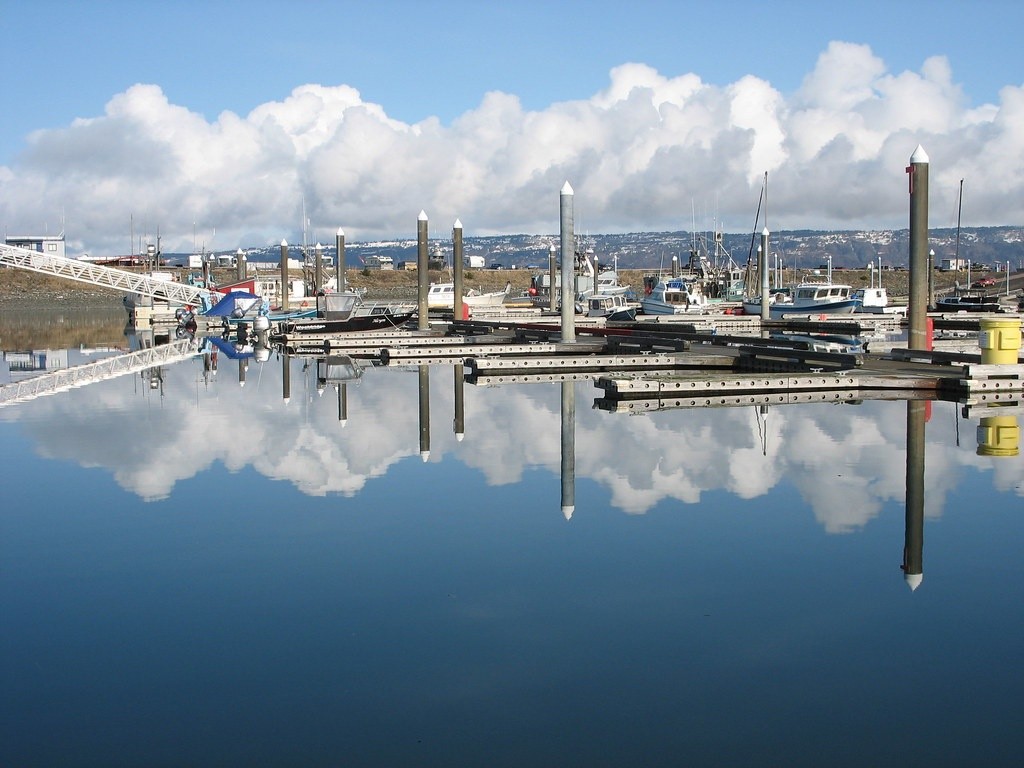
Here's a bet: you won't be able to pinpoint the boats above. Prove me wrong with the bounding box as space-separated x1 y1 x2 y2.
176 292 326 337
120 244 171 325
511 191 908 335
277 285 418 335
428 254 513 309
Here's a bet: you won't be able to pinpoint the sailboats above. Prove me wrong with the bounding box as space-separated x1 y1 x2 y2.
933 177 1002 313
